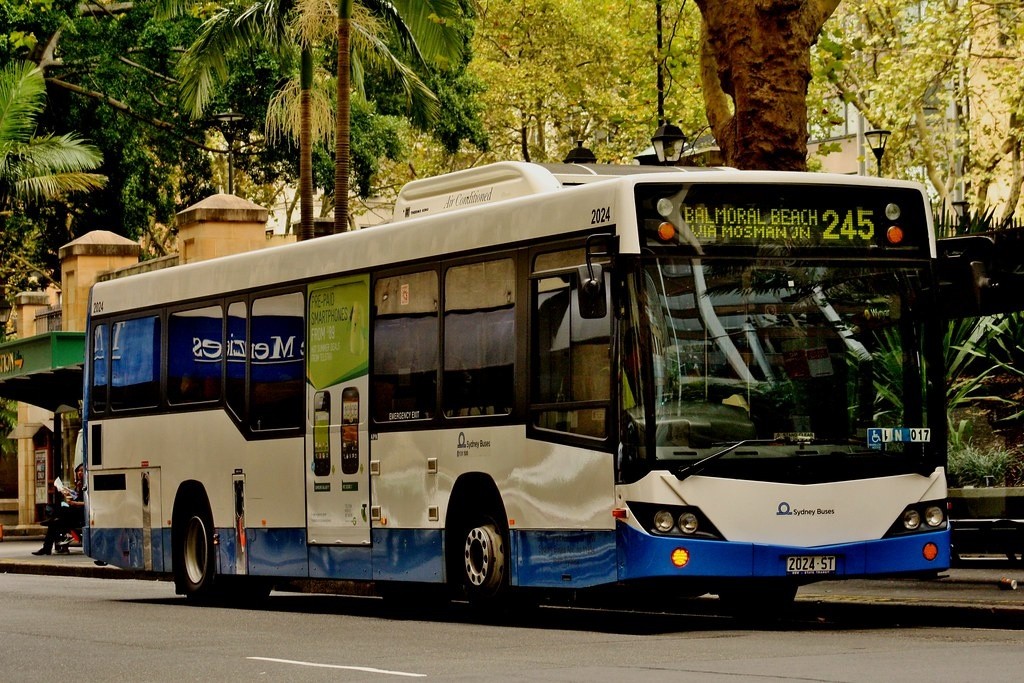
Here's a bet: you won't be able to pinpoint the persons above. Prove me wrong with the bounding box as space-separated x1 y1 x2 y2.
32 463 84 555
591 325 652 436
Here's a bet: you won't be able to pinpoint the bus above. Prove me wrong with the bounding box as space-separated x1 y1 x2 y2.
82 161 1010 626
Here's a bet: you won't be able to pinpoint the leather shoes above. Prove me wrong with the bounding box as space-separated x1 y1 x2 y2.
40 517 59 527
32 547 52 556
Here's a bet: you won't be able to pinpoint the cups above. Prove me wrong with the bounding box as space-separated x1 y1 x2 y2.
997 577 1017 590
